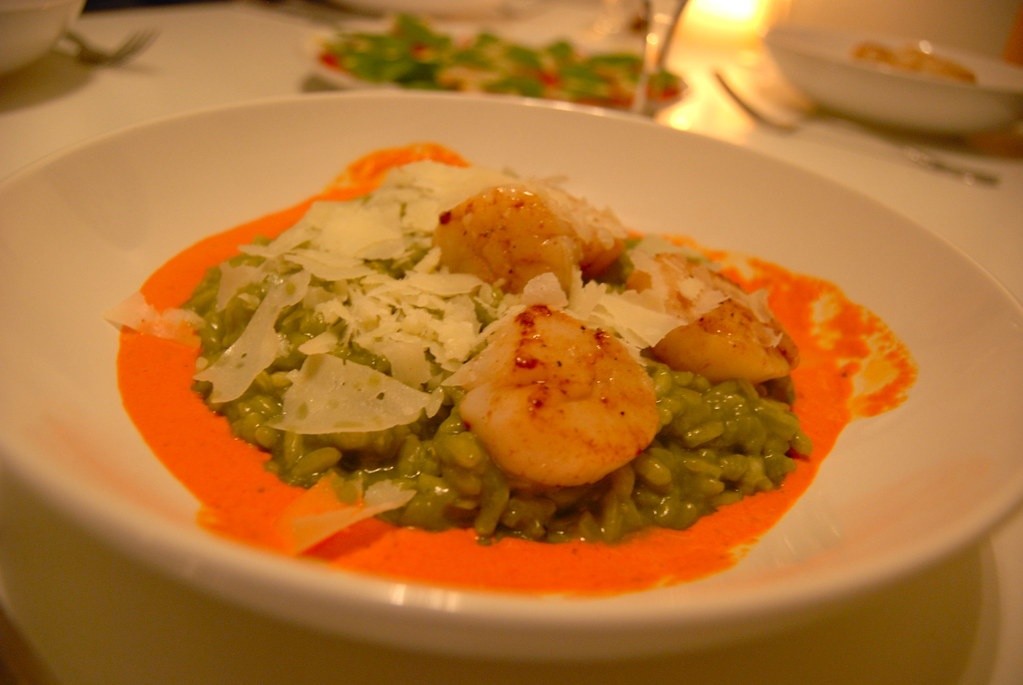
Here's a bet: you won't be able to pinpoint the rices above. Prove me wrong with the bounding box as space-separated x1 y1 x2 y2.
183 236 814 546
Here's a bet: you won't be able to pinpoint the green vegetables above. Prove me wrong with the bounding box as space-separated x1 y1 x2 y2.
328 12 679 103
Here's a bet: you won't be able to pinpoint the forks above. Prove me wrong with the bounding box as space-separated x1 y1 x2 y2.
714 71 1000 189
65 29 161 68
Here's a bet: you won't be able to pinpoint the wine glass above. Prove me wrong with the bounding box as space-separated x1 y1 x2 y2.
631 0 691 117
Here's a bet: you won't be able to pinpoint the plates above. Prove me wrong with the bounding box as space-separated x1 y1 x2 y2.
0 90 1023 663
301 37 688 117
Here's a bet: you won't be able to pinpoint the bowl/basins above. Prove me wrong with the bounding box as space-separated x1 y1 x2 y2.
763 23 1023 134
0 0 85 77
